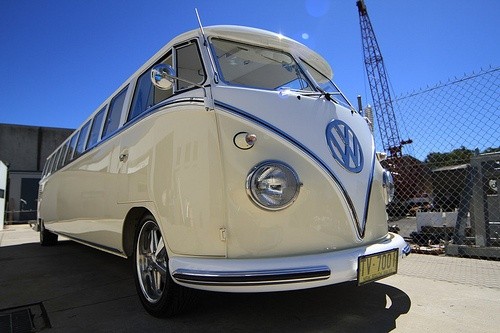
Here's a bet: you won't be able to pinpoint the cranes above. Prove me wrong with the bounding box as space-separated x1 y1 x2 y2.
353 0 423 160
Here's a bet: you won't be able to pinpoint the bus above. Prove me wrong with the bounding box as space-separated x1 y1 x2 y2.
34 25 411 318
